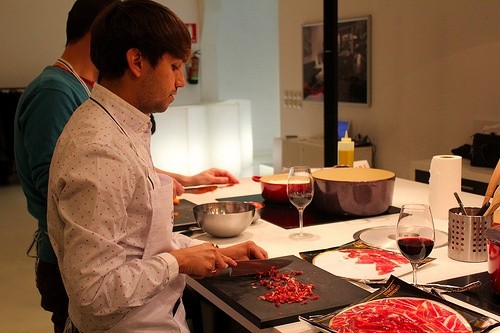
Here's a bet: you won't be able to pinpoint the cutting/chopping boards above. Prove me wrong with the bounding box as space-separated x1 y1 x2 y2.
195 254 372 328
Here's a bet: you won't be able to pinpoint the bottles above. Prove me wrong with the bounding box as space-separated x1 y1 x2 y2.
336 130 354 166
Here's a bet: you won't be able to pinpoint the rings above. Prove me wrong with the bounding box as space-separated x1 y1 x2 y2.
210 270 217 273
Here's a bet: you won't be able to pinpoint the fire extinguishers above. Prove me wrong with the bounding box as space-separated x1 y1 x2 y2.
186 50 202 84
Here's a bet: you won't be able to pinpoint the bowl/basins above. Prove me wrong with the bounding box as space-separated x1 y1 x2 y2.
313 165 396 217
193 200 266 238
252 173 313 205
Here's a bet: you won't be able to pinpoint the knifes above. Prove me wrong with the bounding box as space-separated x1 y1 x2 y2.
222 258 295 277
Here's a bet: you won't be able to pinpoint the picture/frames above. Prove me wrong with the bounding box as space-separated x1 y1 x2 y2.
302 15 371 106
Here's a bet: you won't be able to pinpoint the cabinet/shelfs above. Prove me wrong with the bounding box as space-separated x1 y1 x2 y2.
282 137 372 170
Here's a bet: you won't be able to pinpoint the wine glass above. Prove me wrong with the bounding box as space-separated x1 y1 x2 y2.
286 166 316 241
396 203 436 287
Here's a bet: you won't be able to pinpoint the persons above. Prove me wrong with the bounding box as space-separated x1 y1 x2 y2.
47 0 268 333
17 0 101 333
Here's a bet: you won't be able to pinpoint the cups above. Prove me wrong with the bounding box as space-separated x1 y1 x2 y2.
482 222 500 295
338 121 350 139
